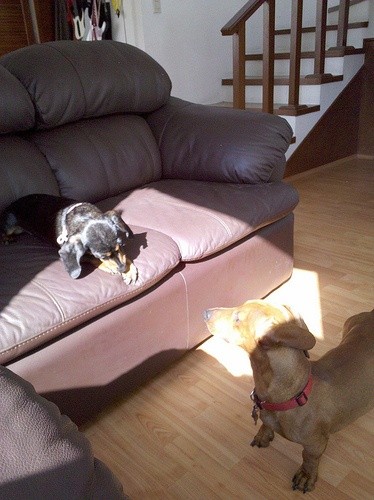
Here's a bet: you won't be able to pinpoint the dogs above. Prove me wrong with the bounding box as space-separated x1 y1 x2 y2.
204 298 374 494
0 192 140 285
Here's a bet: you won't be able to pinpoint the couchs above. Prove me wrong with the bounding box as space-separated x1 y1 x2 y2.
0 40 300 500
